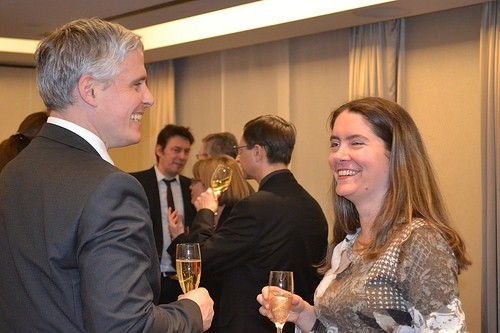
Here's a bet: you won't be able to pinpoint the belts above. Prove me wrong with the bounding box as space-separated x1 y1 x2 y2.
160 272 177 278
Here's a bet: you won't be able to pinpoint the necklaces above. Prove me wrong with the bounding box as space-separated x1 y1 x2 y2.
347 240 358 263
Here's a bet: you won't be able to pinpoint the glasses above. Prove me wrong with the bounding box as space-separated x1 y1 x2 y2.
191 178 201 185
233 144 263 155
196 154 213 160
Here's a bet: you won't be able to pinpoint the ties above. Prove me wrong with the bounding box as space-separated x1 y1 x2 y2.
162 178 176 243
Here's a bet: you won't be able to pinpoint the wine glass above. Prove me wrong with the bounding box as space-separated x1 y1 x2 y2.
211 164 233 200
267 271 294 333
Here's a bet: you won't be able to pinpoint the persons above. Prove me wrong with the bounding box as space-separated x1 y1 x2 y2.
1 111 48 174
187 114 329 333
196 130 257 197
0 17 216 333
256 96 474 333
164 153 252 333
128 123 195 306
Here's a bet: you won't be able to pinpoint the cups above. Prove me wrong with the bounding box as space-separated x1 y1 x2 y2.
176 243 201 294
